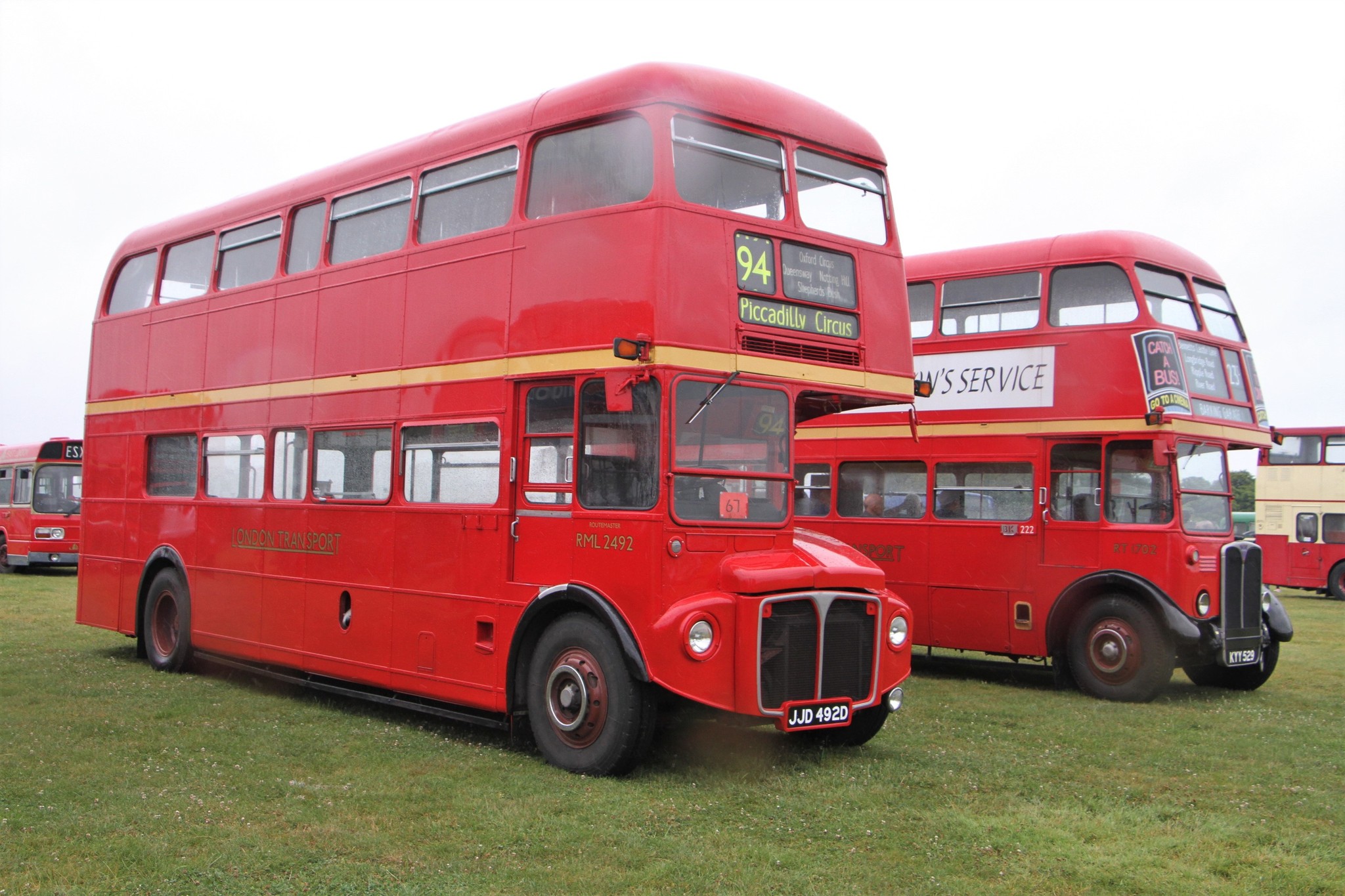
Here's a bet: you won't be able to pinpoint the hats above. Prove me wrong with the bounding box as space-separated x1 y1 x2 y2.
938 490 965 505
904 494 922 503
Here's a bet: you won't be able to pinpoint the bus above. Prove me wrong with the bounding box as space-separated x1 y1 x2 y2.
1255 427 1344 601
0 436 83 575
76 58 932 779
591 229 1294 702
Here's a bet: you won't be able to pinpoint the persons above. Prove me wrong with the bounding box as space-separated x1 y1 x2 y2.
795 488 966 520
